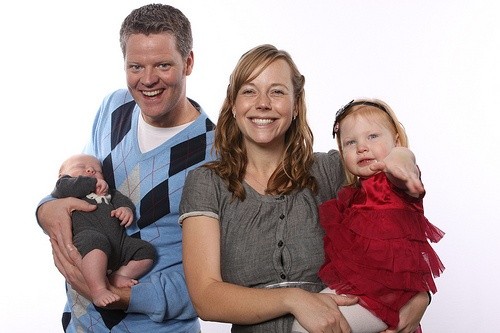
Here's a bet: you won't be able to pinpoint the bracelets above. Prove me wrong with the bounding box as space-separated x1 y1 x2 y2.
427 291 431 305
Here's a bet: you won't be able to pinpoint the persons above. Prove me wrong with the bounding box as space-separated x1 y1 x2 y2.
177 44 432 333
292 98 446 333
51 154 155 307
35 3 220 333
